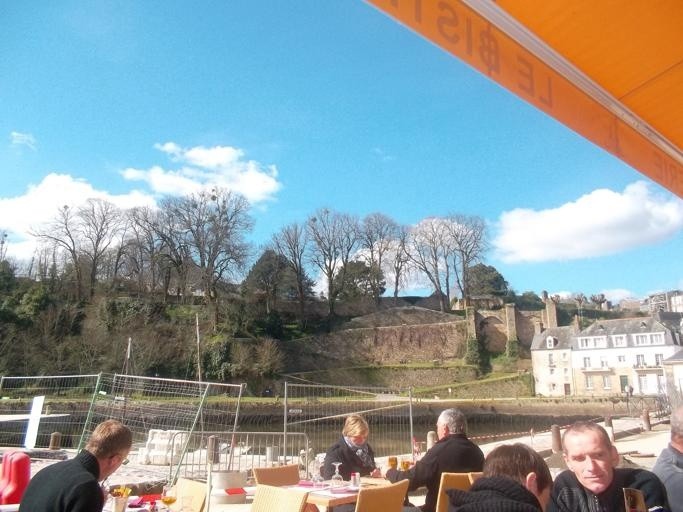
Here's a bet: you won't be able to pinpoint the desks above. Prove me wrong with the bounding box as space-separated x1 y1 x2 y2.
1 477 392 512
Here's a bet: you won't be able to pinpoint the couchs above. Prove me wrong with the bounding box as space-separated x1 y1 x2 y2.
137 428 189 465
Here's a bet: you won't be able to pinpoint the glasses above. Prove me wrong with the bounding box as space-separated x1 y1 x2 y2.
122 457 130 466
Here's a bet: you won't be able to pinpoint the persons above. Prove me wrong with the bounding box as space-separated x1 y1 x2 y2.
323 414 381 512
381 409 485 512
650 403 683 512
20 418 132 511
546 421 668 512
446 441 553 511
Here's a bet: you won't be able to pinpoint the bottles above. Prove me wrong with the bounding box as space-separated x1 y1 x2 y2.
350 471 361 487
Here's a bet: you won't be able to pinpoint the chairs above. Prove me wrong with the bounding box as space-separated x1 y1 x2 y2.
251 463 301 487
164 478 213 511
249 484 310 511
435 471 484 511
1 449 31 505
355 477 411 511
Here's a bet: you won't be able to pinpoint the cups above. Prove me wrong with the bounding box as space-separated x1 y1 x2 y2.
108 494 130 512
388 456 397 469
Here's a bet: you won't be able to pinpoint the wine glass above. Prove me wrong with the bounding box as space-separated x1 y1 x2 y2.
330 461 344 488
312 462 324 488
161 493 177 512
400 457 410 471
